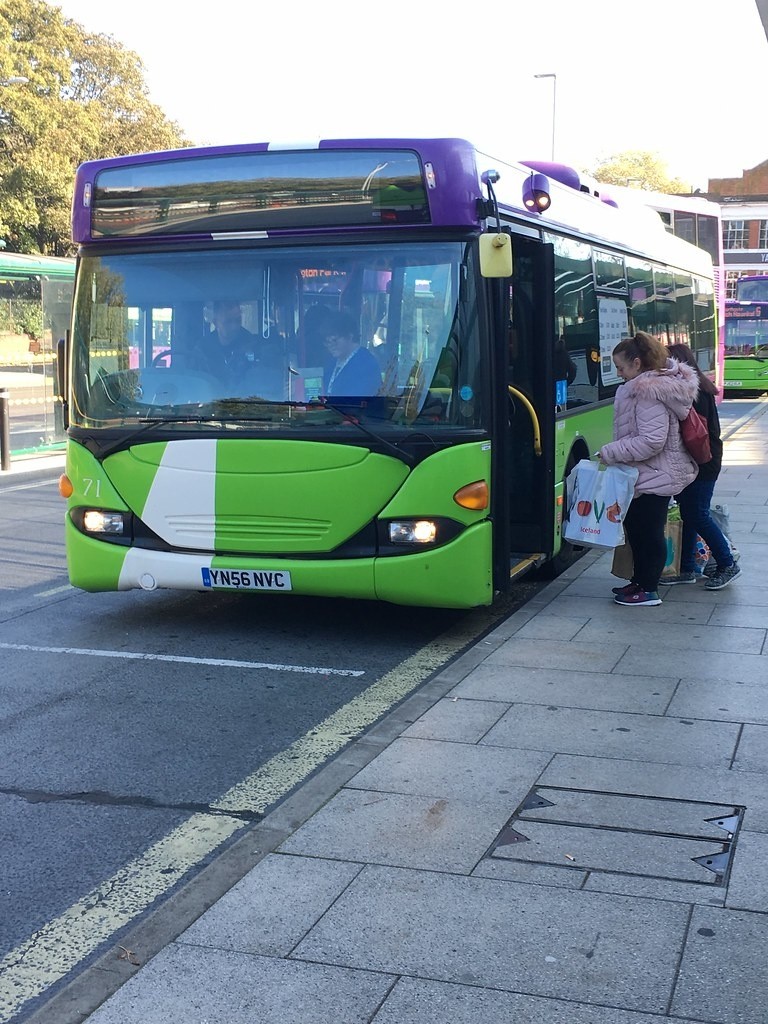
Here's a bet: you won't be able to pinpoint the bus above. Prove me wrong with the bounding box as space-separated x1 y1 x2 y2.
119 160 768 415
46 137 718 611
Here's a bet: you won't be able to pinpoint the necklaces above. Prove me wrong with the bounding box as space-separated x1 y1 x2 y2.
326 347 362 395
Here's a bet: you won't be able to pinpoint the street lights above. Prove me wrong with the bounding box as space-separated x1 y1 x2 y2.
534 72 557 166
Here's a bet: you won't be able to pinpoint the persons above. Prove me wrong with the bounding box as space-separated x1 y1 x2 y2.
599 331 700 606
659 344 742 590
186 295 383 397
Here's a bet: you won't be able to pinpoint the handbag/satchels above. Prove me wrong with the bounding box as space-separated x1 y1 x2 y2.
678 403 713 465
610 525 634 580
561 458 640 550
661 514 683 577
694 503 741 575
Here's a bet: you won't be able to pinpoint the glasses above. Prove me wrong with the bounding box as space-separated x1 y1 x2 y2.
323 334 346 347
217 315 241 326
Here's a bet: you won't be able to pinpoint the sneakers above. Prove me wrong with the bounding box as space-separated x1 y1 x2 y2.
612 582 641 595
614 585 663 606
705 561 742 589
659 570 697 585
